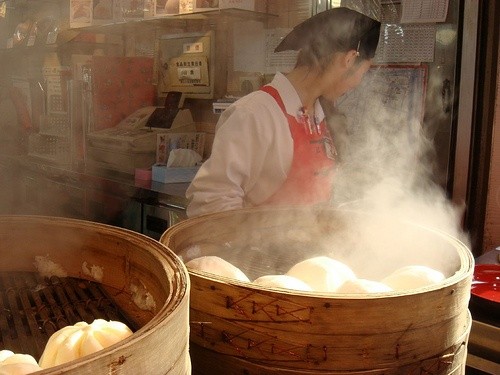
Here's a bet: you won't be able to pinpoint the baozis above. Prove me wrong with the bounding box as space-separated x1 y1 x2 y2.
0 350 43 375
38 319 134 369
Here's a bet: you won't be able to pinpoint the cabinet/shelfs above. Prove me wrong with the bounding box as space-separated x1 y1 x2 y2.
0 156 191 243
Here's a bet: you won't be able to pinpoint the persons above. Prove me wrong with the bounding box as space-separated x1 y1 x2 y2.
186 8 381 219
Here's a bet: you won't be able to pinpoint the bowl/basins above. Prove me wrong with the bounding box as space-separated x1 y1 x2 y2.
470 263 500 323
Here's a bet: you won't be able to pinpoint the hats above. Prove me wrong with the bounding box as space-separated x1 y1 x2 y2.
274 8 382 52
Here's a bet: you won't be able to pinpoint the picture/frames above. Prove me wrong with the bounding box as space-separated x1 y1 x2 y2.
369 63 430 120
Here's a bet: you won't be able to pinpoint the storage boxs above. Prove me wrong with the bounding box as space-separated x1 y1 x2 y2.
135 167 153 182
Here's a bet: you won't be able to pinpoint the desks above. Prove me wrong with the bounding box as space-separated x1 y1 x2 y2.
471 246 500 327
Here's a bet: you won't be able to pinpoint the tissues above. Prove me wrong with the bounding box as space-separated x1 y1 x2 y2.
152 149 203 183
